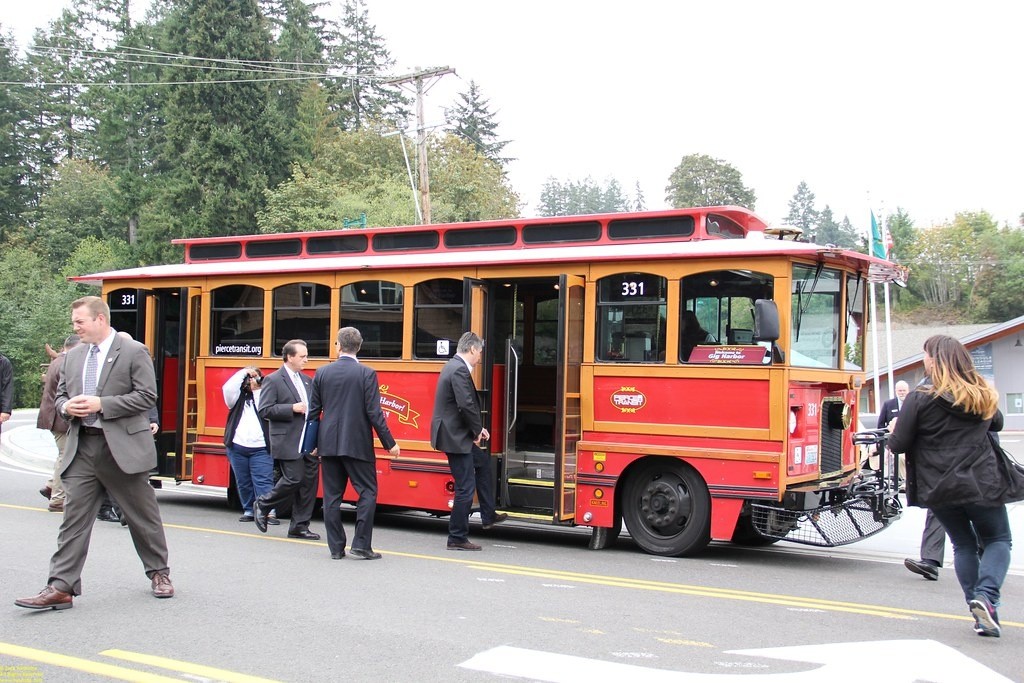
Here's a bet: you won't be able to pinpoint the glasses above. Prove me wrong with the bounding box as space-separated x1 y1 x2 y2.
335 342 338 346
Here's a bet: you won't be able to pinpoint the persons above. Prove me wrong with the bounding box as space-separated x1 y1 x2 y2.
680 309 717 361
887 335 1024 638
0 354 13 447
430 331 508 551
14 295 174 610
97 331 160 526
222 366 280 525
904 508 985 581
307 326 400 560
36 334 81 510
254 339 321 540
878 380 910 493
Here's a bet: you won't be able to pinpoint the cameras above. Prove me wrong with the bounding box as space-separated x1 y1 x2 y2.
254 376 264 384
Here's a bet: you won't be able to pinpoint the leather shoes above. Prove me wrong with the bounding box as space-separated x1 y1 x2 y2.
239 515 255 522
152 573 173 597
904 558 938 581
253 501 267 533
266 516 280 525
120 513 128 526
350 546 381 559
97 509 119 521
13 584 73 610
287 530 320 541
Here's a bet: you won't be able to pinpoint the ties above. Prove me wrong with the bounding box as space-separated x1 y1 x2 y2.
83 346 99 426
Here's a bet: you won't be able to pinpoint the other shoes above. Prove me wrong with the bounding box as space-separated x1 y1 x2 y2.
48 502 63 513
40 485 51 501
899 484 906 493
331 550 345 559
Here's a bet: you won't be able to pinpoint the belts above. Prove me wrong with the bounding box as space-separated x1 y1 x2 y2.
80 425 104 435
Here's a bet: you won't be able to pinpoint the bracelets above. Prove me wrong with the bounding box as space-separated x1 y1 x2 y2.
61 403 68 417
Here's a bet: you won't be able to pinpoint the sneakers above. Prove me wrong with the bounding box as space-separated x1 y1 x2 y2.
973 621 985 634
482 513 508 529
447 540 482 551
968 595 1001 637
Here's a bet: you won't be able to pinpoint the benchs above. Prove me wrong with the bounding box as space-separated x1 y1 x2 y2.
518 363 555 414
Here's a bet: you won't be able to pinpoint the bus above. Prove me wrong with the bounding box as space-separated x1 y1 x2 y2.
67 205 910 558
